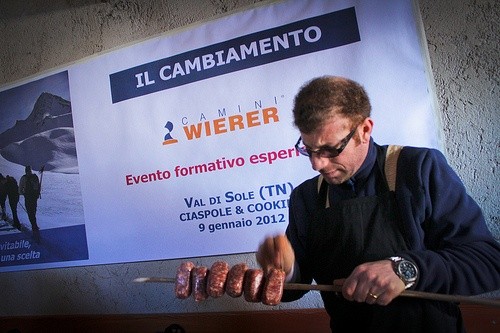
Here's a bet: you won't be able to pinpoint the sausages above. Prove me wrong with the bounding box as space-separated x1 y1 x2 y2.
176 260 285 306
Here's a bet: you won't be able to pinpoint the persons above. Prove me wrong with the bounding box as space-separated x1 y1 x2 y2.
19 166 40 231
0 173 8 221
6 175 20 226
255 75 500 333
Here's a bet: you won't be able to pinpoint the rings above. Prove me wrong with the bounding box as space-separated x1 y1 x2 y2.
369 293 378 299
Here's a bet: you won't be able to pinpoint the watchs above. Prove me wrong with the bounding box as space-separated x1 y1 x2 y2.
386 256 418 288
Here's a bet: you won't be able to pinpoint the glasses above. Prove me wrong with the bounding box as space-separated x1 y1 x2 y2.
295 130 356 158
